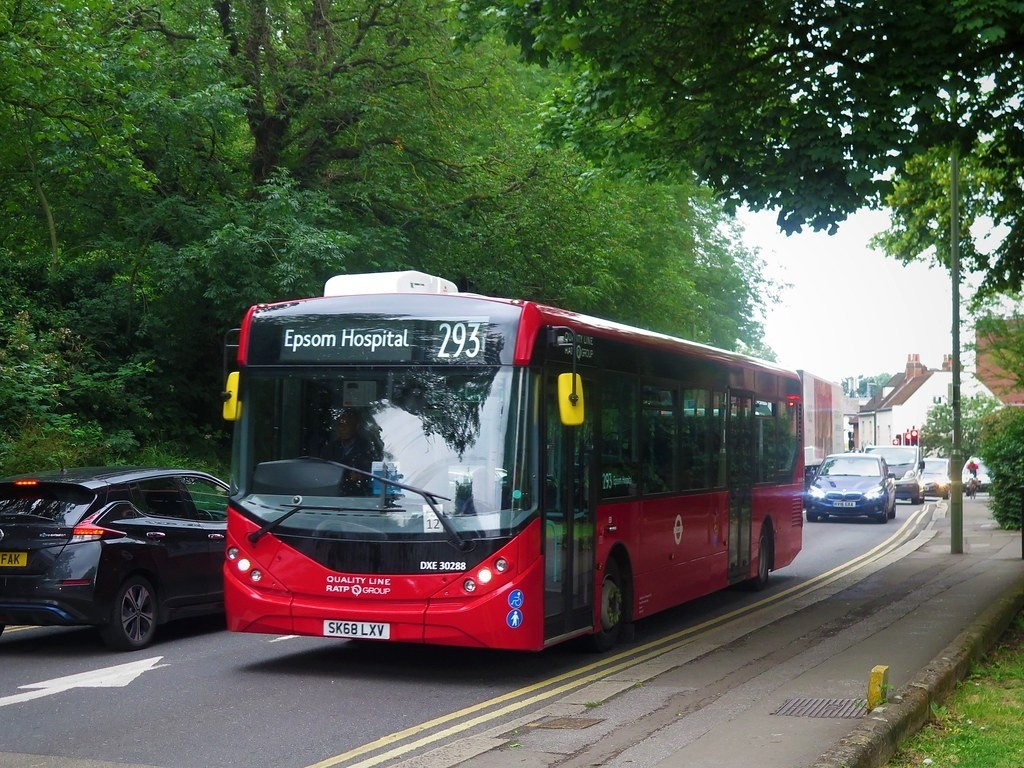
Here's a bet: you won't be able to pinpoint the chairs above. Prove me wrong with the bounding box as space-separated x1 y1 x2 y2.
603 427 788 497
142 486 185 519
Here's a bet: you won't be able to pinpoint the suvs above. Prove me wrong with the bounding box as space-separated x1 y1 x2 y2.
861 445 926 505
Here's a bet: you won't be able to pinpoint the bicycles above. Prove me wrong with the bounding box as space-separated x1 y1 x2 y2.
965 475 981 500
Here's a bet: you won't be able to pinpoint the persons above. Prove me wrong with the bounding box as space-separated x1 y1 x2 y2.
966 462 980 491
317 407 382 497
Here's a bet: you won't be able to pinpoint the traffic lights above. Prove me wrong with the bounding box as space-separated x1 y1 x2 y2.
903 433 911 446
893 438 899 446
910 430 919 446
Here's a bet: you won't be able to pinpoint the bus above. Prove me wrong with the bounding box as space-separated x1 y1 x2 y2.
218 269 806 655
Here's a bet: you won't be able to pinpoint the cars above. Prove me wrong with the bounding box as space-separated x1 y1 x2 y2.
923 458 953 500
0 462 238 651
804 452 898 525
